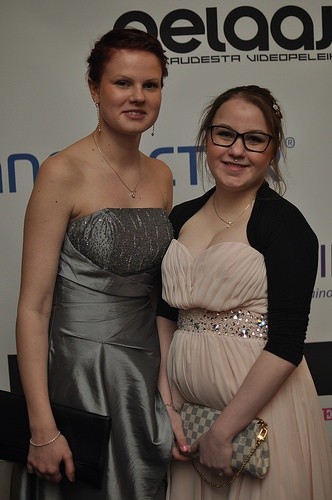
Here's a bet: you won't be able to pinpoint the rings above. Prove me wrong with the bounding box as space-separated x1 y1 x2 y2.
220 472 224 478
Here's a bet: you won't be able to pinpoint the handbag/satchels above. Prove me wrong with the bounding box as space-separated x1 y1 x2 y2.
179 403 270 487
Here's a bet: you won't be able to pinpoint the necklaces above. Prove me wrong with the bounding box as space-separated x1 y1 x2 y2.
212 195 253 228
91 134 141 199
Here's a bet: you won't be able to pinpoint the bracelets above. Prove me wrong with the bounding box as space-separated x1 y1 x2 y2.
29 431 60 447
164 403 175 412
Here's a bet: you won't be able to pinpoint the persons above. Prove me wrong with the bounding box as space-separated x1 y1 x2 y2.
10 28 175 500
155 84 332 500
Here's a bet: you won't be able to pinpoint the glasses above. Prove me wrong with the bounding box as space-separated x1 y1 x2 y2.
209 125 273 152
